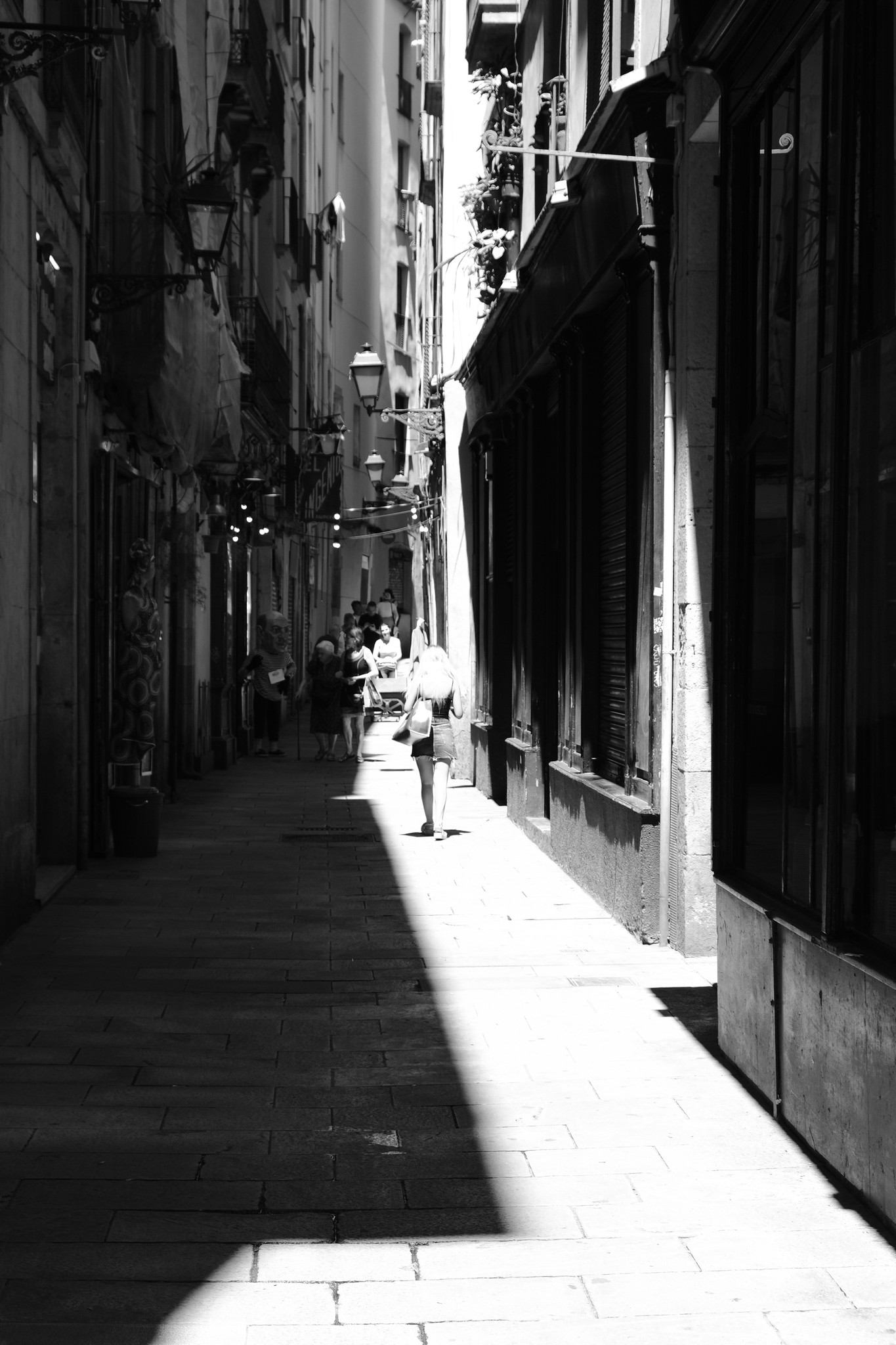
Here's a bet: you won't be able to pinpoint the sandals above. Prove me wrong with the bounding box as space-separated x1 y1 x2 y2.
315 748 327 760
356 755 363 762
339 750 356 762
327 752 336 761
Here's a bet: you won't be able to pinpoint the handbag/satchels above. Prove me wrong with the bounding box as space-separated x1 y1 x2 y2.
363 674 383 708
392 676 432 746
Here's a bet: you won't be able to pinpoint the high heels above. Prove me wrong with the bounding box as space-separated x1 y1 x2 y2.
421 822 434 835
433 825 448 840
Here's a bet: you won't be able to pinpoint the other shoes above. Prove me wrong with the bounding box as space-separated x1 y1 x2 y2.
267 749 286 756
254 749 268 756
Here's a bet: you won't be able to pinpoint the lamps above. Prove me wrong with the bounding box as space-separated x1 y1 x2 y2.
348 341 445 446
82 167 238 326
366 450 438 516
276 412 347 498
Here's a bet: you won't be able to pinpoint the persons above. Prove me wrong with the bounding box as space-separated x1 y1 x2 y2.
403 646 464 840
294 590 402 763
234 611 297 759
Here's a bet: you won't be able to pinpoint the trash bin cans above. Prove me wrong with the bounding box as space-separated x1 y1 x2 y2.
107 786 161 855
